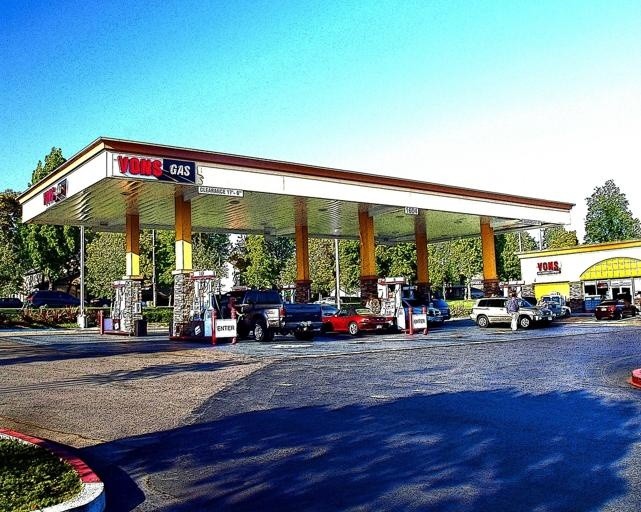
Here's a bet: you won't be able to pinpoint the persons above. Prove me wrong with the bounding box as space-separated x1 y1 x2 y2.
509 292 519 331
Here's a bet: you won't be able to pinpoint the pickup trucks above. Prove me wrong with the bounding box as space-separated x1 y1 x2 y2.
218 291 322 342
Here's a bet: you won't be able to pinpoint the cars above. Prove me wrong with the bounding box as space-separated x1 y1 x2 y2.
470 291 571 329
27 290 111 309
402 298 451 329
0 297 22 308
594 300 639 320
445 287 484 299
314 296 396 335
